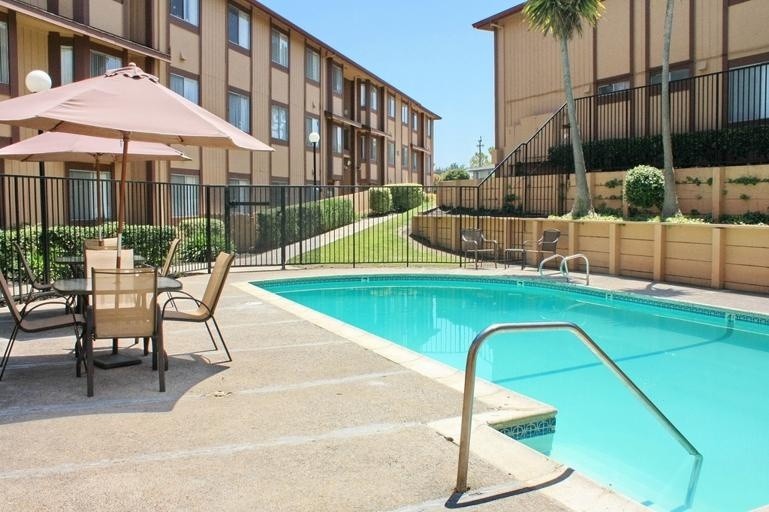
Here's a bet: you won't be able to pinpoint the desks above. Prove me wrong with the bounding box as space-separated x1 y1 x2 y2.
46 249 147 276
502 247 528 271
49 274 184 372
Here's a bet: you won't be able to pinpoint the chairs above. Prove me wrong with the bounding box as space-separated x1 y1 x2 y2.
459 226 499 270
81 237 124 246
2 268 94 385
76 266 170 394
152 251 240 372
133 236 184 356
516 226 561 273
78 247 141 347
9 236 85 319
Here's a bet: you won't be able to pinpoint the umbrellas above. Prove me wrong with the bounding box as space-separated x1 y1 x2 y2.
0 131 192 244
0 62 277 355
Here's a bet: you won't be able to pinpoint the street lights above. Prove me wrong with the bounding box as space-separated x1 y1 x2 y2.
309 132 320 202
26 70 52 300
